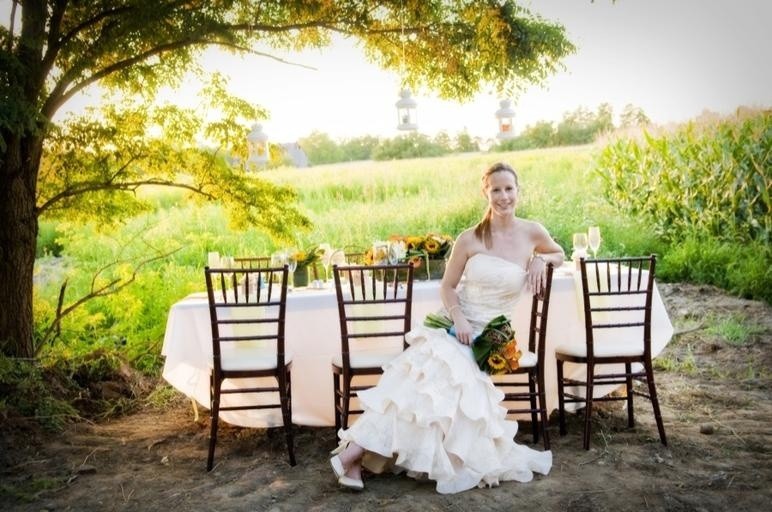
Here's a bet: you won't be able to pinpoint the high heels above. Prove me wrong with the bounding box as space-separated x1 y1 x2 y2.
330 439 349 477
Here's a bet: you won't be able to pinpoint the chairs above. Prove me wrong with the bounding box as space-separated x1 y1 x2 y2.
332 262 413 447
225 257 279 285
493 262 554 451
204 265 296 472
313 253 367 278
555 254 667 450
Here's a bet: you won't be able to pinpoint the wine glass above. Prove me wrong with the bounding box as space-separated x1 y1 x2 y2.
588 225 600 259
207 238 406 295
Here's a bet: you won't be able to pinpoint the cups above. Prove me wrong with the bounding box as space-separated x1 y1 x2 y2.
572 250 588 271
573 233 588 252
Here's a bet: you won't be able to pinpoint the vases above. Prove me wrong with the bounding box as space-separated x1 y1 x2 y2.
294 269 308 288
375 259 446 282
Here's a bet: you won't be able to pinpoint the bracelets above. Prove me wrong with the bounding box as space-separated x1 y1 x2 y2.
531 256 547 263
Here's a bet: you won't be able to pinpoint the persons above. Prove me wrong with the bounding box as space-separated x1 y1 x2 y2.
329 163 565 494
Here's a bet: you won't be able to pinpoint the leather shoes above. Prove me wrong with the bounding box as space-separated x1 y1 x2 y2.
338 475 364 489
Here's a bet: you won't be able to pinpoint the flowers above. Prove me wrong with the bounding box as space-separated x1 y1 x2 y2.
364 230 453 269
286 243 325 271
423 313 522 378
